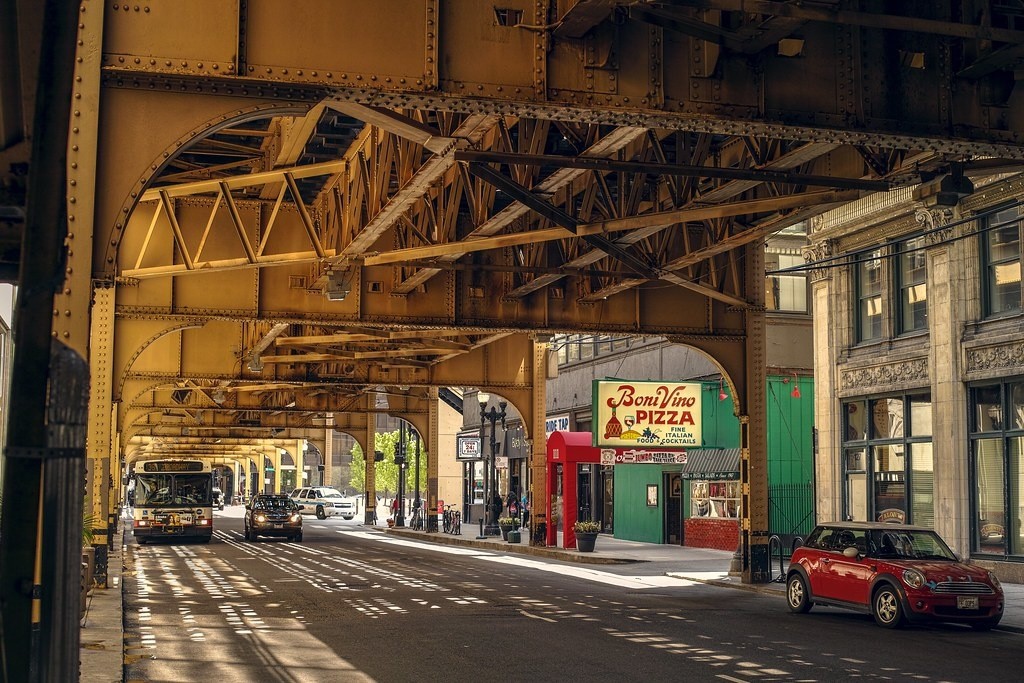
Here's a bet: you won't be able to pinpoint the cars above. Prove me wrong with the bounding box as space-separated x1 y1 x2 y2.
786 522 1004 632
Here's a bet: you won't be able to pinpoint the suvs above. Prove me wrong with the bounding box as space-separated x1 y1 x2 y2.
286 485 356 521
244 493 304 544
212 487 225 511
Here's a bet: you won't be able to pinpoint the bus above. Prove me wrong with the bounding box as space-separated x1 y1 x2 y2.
134 457 213 544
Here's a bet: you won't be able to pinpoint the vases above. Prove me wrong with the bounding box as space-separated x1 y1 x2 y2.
501 526 519 541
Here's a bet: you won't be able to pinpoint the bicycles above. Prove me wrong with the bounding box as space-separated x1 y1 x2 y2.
440 504 463 535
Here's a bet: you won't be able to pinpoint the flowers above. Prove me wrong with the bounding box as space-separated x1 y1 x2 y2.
571 520 600 533
497 517 521 524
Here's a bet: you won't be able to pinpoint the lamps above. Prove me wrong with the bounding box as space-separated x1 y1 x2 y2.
789 370 800 397
720 377 728 401
912 161 975 210
247 354 264 372
213 392 226 404
322 280 351 301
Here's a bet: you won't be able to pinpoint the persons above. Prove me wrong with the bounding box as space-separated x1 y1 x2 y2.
392 494 422 523
146 482 159 501
188 485 201 504
486 491 530 531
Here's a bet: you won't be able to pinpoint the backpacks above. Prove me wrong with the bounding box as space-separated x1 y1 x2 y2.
494 499 502 512
510 500 517 513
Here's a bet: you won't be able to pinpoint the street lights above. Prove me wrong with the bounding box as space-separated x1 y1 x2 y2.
478 390 508 536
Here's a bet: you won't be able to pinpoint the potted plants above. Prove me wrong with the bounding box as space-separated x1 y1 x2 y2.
575 533 598 552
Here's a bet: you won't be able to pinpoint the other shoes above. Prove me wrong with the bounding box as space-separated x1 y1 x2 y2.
521 527 523 531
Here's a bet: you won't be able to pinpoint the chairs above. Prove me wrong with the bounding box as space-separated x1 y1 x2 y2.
882 534 901 554
820 531 868 551
